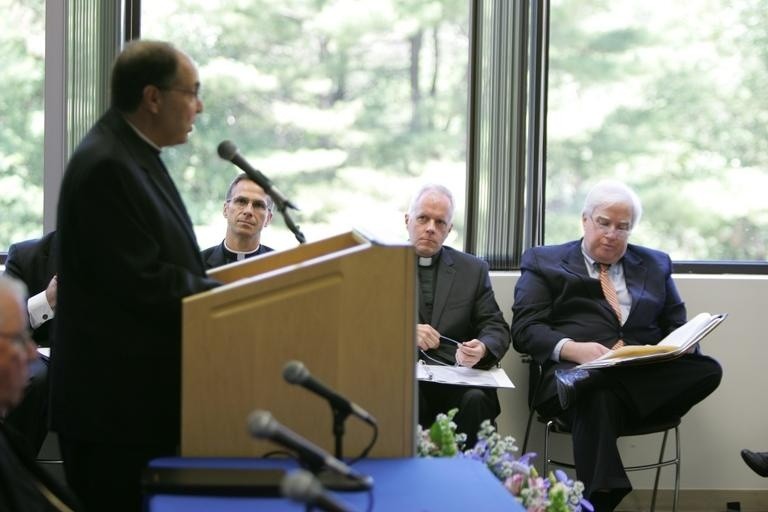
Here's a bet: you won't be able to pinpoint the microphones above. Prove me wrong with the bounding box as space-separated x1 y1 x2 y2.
217 140 287 212
275 466 354 512
244 408 372 481
279 359 372 422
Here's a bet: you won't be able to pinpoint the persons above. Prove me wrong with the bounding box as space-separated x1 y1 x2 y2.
50 38 223 511
403 182 513 453
741 449 767 479
4 229 67 464
0 274 59 509
198 173 275 270
508 180 723 512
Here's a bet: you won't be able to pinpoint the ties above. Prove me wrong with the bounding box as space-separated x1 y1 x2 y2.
597 263 625 350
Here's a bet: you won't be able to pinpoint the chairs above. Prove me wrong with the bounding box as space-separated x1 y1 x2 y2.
521 356 682 511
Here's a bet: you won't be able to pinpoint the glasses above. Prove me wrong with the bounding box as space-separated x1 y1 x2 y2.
588 213 635 238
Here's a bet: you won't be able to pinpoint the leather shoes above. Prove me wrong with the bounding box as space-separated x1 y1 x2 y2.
550 367 589 411
738 447 768 477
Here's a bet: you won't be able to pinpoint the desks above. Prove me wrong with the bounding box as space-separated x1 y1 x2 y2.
145 449 526 512
